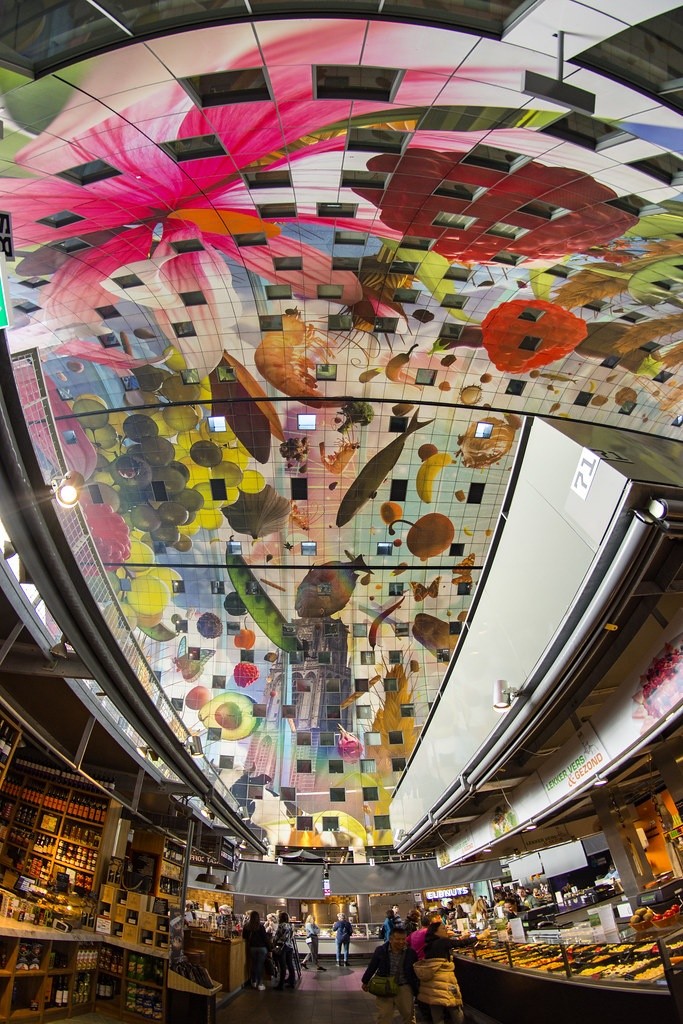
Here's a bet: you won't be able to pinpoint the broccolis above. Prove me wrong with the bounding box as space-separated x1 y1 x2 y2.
338 401 373 434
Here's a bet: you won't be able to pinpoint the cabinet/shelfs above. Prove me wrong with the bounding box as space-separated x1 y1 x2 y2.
0 710 187 1024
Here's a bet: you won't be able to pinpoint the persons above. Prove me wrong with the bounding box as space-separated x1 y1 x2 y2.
361 864 624 1024
184 899 353 991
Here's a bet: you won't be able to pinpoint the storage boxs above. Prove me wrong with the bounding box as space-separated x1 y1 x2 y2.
616 901 634 918
621 894 629 901
0 842 4 854
3 870 19 889
0 823 8 839
0 888 17 918
15 876 35 892
29 942 44 970
40 814 60 832
16 943 31 971
587 902 617 937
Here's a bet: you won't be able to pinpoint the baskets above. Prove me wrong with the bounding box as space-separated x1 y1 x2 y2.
651 912 680 929
628 920 652 931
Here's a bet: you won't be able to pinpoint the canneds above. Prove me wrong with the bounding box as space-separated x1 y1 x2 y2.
56 840 98 871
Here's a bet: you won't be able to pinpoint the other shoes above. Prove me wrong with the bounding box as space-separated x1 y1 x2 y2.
284 985 294 988
300 963 309 969
344 962 350 967
336 962 341 967
258 983 266 990
272 986 283 990
251 980 257 987
317 967 326 970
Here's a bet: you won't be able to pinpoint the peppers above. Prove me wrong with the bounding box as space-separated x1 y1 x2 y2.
386 343 419 383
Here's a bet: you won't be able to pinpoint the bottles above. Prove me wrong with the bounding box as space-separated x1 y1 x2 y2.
153 959 164 986
33 833 57 857
1 719 116 827
63 977 69 1006
96 976 113 999
13 981 17 1000
161 877 178 896
54 977 63 1007
164 841 183 864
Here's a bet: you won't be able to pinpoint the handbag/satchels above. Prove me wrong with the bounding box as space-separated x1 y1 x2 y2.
379 926 386 939
273 946 282 955
366 975 398 997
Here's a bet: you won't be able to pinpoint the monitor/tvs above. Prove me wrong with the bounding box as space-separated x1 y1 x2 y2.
14 875 36 893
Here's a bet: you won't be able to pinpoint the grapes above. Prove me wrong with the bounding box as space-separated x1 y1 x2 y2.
64 346 266 639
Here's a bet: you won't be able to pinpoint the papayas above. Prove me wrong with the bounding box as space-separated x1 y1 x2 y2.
198 692 262 741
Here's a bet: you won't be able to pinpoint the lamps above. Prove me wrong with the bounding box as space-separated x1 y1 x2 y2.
239 805 250 821
13 736 26 748
208 813 215 820
214 854 237 892
187 735 204 756
264 837 271 847
50 643 67 658
493 680 511 713
527 819 537 830
174 803 193 817
55 470 84 509
595 773 608 786
136 747 147 757
195 865 222 884
149 750 159 761
395 829 404 841
3 540 16 560
239 840 247 849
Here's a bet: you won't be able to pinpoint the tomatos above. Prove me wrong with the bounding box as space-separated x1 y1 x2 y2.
652 905 679 920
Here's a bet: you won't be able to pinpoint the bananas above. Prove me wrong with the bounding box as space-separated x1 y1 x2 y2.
416 453 457 503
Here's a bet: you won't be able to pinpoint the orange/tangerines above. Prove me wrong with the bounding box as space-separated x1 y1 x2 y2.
407 513 454 560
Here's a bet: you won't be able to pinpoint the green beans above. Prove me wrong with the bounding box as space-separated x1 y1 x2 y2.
226 535 302 651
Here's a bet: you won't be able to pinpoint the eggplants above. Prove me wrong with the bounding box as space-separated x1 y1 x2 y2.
428 326 484 355
